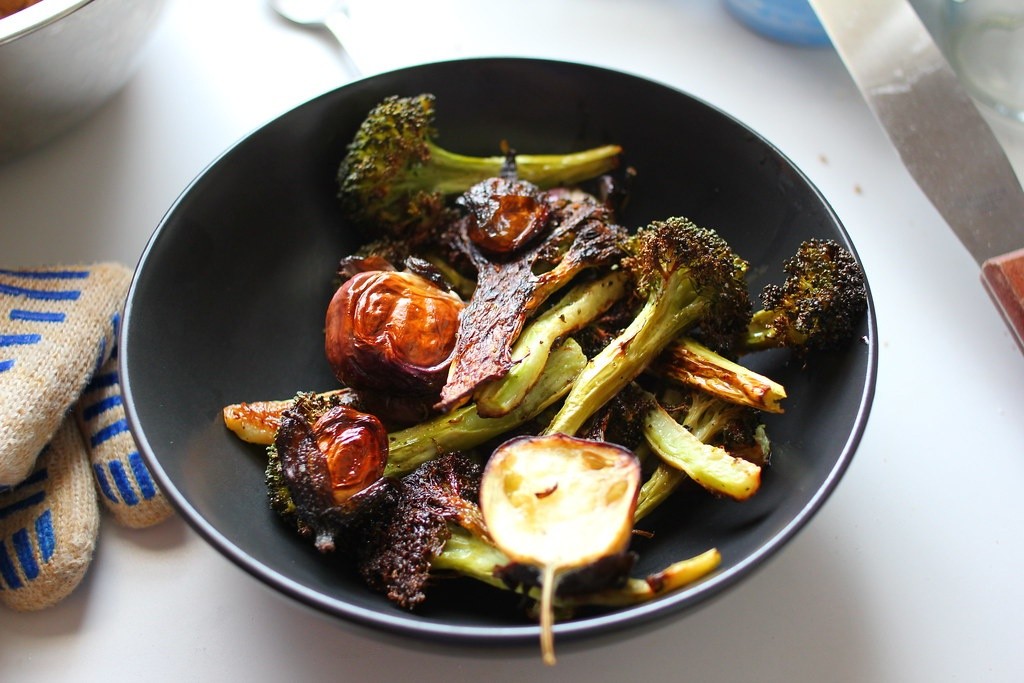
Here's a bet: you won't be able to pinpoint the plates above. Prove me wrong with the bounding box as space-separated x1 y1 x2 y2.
117 57 877 644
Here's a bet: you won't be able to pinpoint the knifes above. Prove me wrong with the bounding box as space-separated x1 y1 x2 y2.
808 0 1024 359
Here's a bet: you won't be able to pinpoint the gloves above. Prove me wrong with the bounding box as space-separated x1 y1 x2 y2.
0 263 176 610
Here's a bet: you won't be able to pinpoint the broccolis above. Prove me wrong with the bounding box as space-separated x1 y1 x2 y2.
265 94 868 616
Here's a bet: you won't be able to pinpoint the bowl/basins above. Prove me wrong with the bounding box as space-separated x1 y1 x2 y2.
0 2 170 154
